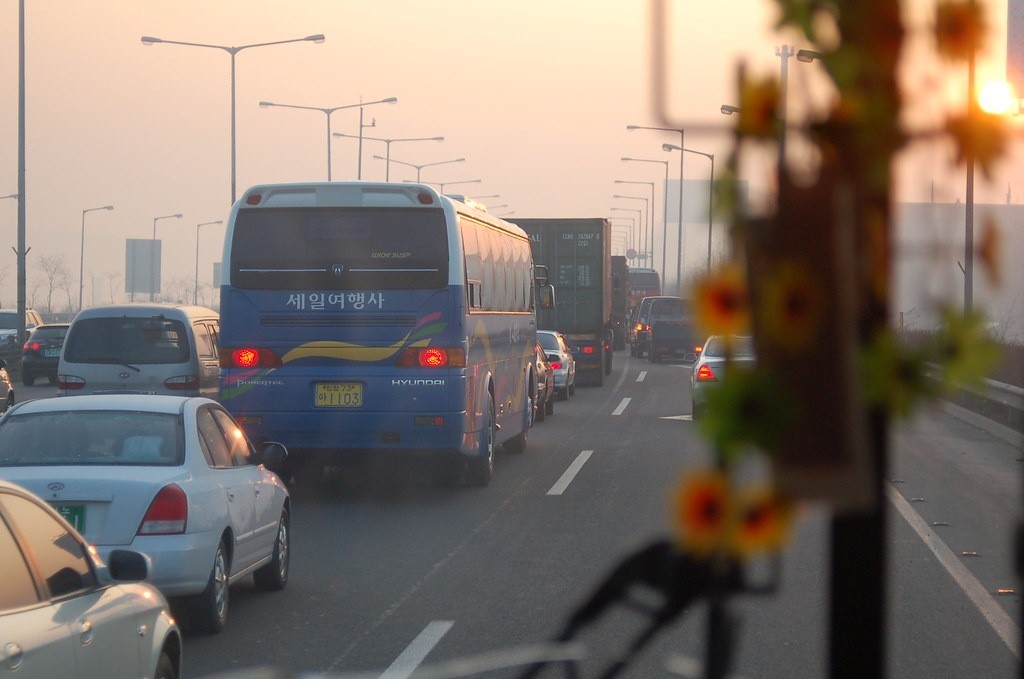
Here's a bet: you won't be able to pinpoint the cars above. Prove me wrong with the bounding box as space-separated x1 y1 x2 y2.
0 477 184 678
535 330 581 402
0 393 292 634
0 358 15 413
689 333 756 423
536 340 560 422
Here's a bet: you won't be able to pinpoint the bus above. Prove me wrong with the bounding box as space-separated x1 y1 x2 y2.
215 182 555 488
626 267 661 315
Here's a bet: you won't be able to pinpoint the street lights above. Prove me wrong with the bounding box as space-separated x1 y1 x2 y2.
661 143 713 277
372 154 466 184
150 213 186 302
79 206 114 313
141 34 326 207
258 96 399 183
607 216 636 267
194 220 224 306
614 180 655 269
610 207 642 268
403 179 481 196
626 125 685 297
331 132 444 182
621 157 669 297
613 194 648 268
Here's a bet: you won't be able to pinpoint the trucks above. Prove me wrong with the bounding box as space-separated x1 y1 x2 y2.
609 255 629 352
500 216 620 387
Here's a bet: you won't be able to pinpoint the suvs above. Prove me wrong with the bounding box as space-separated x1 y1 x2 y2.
21 324 70 386
627 296 704 365
0 309 43 350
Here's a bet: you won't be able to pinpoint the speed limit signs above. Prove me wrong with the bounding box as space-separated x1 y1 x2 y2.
626 249 637 260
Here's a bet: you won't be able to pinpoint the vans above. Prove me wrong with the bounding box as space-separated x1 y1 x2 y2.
58 300 221 399
